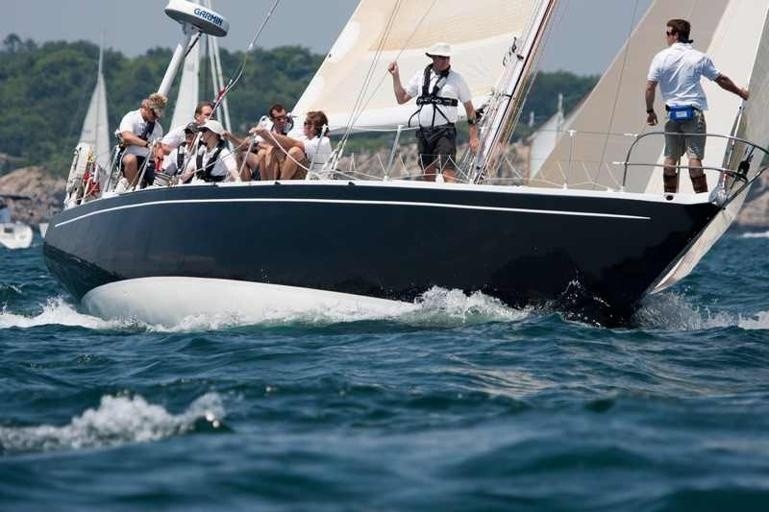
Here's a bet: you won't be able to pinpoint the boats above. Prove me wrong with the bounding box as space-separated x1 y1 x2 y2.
0 194 35 251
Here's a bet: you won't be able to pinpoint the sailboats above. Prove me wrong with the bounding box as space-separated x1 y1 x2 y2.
43 0 765 328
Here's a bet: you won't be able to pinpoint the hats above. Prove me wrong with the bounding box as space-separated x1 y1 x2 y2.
184 123 201 134
197 120 225 141
148 104 165 118
426 43 450 57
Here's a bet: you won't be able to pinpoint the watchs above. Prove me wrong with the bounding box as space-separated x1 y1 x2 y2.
467 118 478 124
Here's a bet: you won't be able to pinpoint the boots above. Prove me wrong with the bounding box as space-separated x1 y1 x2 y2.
663 173 677 193
690 174 707 193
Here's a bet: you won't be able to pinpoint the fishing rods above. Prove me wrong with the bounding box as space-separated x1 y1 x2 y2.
175 1 282 184
319 0 435 178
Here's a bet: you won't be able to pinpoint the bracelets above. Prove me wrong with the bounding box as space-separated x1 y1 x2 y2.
145 142 150 148
648 108 654 113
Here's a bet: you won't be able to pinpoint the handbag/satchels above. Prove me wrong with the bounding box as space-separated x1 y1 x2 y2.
668 106 693 123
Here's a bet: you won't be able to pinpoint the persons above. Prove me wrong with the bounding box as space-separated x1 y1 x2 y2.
249 111 334 180
645 20 750 200
387 42 479 183
153 101 215 187
115 93 168 190
178 119 242 184
221 104 303 180
